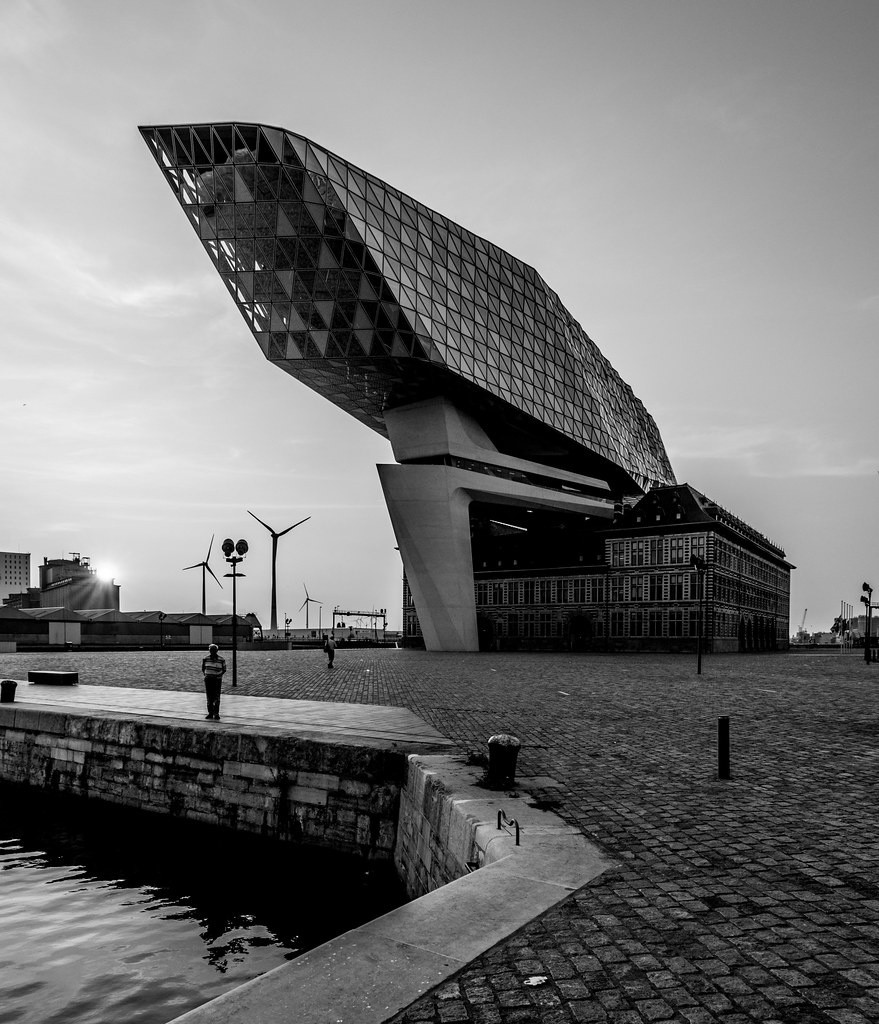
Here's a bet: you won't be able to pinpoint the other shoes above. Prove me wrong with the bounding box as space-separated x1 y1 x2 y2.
205 713 213 719
213 713 220 720
328 664 333 668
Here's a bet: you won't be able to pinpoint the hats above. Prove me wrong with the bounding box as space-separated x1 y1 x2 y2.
208 644 218 650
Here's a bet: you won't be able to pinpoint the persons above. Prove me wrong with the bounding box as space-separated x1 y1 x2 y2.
325 635 337 669
201 644 226 720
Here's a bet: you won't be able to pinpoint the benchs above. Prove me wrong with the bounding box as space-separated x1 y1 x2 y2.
28 671 78 687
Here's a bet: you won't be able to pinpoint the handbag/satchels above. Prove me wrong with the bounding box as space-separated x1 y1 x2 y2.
324 640 330 652
